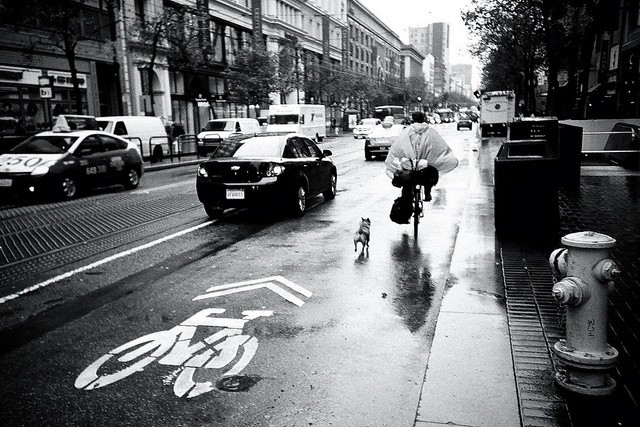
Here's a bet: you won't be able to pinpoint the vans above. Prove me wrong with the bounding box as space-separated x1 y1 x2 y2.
94 115 169 163
196 117 260 157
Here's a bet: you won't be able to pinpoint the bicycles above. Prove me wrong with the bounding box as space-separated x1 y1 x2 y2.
411 182 424 238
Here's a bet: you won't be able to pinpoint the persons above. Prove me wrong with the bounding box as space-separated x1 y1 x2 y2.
385 111 459 203
165 119 187 156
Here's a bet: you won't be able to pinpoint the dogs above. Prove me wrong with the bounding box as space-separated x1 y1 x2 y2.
354 217 371 255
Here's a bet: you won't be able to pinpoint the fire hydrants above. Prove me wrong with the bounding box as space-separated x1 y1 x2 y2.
547 230 623 402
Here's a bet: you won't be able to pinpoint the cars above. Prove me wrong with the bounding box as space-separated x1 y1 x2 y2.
457 119 472 130
365 123 407 161
196 132 337 219
353 118 382 139
0 114 145 200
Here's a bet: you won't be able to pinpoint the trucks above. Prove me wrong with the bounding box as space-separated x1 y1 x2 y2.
477 89 516 137
266 104 326 143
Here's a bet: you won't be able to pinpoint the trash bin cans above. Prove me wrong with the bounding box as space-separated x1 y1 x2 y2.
493 139 561 251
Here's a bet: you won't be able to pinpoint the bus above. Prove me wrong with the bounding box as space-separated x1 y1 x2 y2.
374 105 404 121
